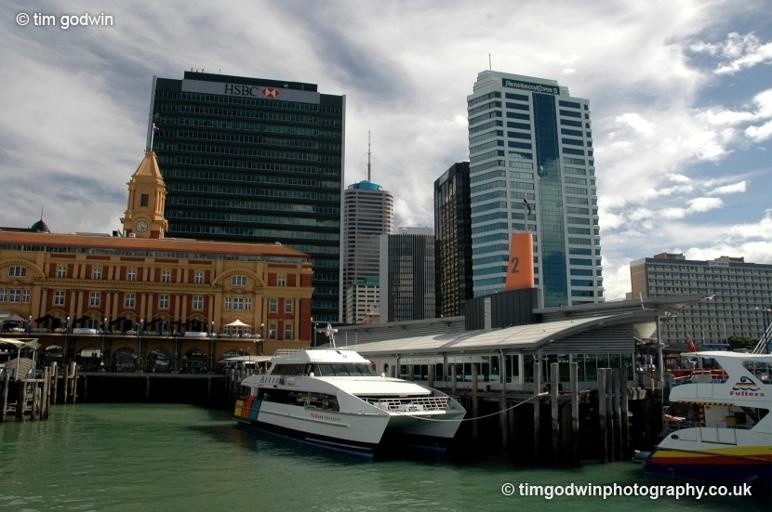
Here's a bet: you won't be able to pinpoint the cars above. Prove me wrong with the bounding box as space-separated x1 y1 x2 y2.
635 353 656 373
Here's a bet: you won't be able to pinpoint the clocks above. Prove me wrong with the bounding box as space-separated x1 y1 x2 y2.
132 218 149 236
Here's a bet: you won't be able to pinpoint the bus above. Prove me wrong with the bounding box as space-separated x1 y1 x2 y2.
664 351 727 381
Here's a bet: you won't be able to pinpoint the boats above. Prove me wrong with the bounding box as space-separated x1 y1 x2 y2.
631 320 772 488
230 316 472 462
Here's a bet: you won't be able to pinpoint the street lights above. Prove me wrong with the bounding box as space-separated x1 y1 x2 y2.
754 306 772 355
308 316 317 347
27 311 268 374
